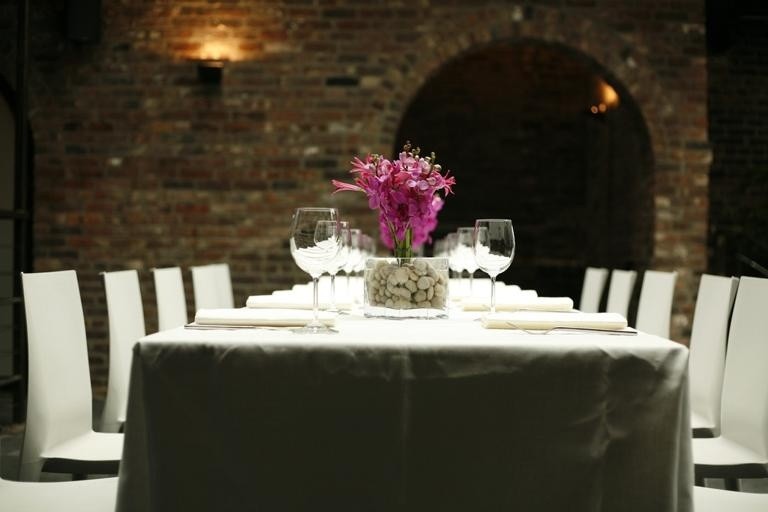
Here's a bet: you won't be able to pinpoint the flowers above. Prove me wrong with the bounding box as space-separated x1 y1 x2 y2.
328 138 457 265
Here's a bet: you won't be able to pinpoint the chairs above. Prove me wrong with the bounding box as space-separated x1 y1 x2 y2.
16 268 125 481
187 262 235 310
149 264 189 333
1 469 120 510
607 268 638 318
98 267 148 433
633 268 680 339
690 274 768 493
692 484 767 512
577 266 610 314
686 273 740 437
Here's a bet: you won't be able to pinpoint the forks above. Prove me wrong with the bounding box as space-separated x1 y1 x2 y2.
507 320 639 335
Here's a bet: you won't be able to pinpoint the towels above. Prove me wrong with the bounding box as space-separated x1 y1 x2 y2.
194 275 366 328
453 276 630 330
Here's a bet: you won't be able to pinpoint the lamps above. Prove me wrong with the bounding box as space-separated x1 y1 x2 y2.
196 57 226 86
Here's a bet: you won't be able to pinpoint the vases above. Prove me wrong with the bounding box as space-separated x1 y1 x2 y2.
362 255 450 315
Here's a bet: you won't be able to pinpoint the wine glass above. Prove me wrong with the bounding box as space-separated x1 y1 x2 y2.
290 207 518 335
